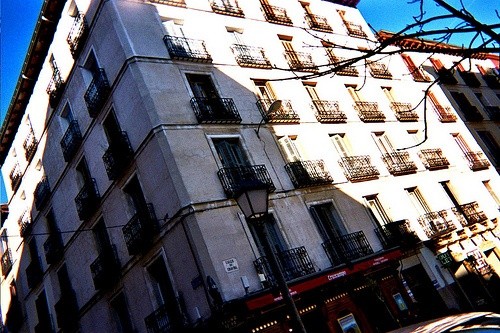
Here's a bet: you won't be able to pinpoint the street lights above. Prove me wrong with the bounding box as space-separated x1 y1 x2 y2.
231 176 308 332
